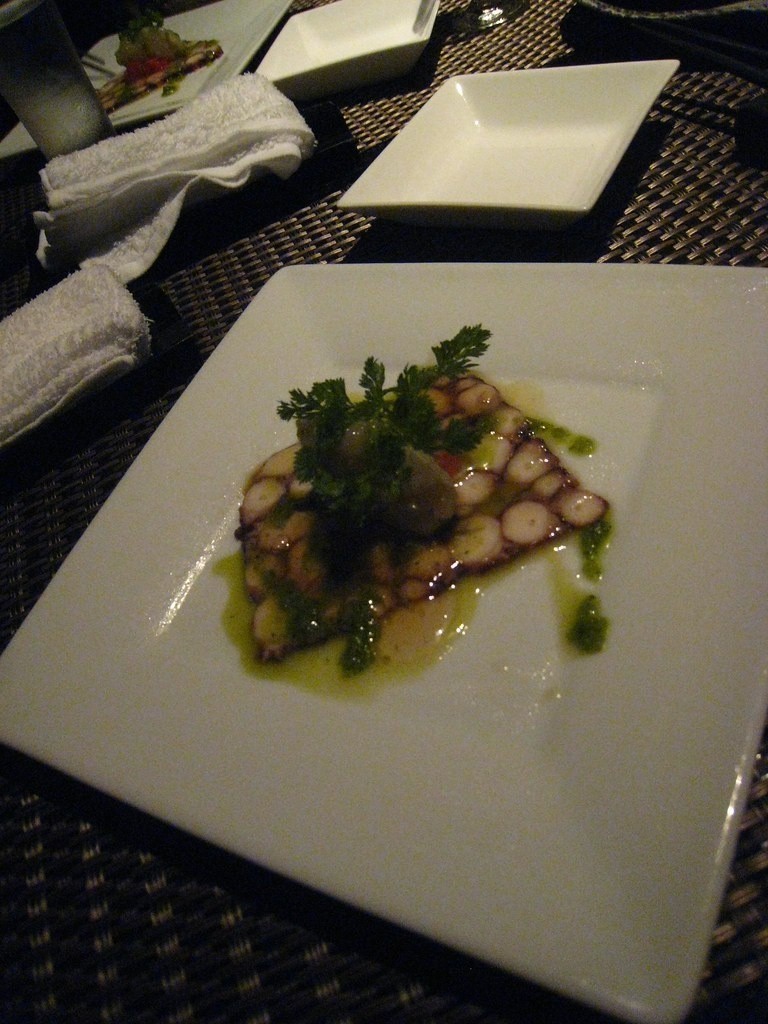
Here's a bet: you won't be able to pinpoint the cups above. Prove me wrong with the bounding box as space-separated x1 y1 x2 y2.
0 0 114 163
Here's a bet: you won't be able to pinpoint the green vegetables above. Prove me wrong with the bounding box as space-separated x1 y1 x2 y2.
276 322 493 534
118 10 164 40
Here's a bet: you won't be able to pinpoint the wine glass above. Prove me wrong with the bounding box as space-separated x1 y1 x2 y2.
453 0 527 33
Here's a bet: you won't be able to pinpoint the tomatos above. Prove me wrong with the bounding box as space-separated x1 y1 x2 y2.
125 58 166 79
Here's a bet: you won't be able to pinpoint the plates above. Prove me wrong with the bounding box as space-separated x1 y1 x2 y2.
571 0 768 21
0 0 300 158
337 59 680 229
0 265 768 1024
256 0 441 103
